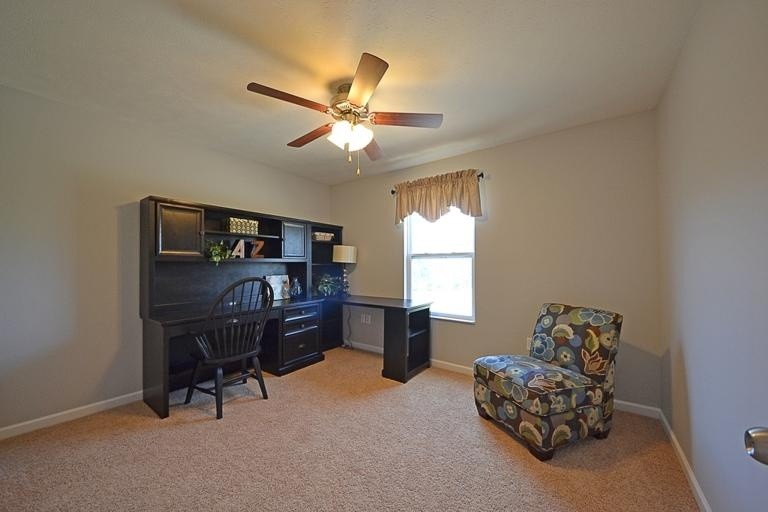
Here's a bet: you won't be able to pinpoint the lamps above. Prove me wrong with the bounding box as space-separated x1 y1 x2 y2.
332 244 357 295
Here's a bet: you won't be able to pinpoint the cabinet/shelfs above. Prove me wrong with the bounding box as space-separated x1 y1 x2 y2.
140 196 344 419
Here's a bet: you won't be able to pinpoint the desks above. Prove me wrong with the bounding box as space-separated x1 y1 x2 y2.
142 296 433 418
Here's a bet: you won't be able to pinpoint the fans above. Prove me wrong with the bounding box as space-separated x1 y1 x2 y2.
247 52 444 162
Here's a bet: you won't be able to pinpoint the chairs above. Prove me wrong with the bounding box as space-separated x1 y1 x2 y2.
473 301 624 462
184 277 274 418
